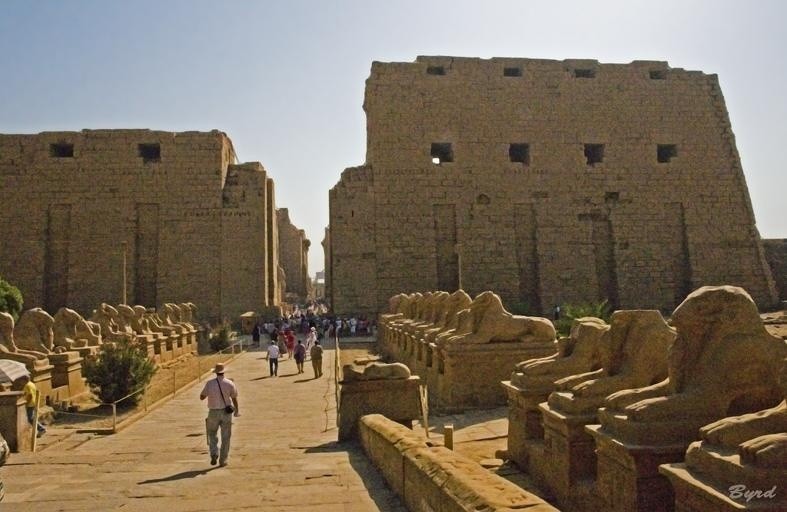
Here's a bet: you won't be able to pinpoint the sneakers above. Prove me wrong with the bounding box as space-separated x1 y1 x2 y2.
211 455 227 467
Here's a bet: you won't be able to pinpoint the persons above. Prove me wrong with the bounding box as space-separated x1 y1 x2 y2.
310 340 323 379
251 312 371 347
294 340 307 374
266 340 280 376
287 331 295 358
278 331 287 358
22 375 46 438
200 363 241 467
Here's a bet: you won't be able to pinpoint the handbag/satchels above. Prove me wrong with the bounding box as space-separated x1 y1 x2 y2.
294 353 300 360
225 406 234 413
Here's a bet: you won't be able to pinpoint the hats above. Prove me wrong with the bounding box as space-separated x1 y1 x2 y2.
211 363 228 373
